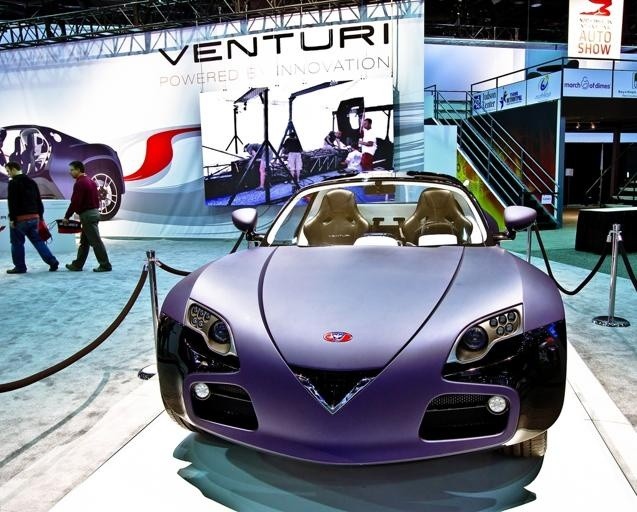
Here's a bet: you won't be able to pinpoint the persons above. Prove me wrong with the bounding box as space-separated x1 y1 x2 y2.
243 143 273 191
323 130 344 148
356 117 377 173
4 161 60 274
61 160 112 272
344 143 362 174
284 131 303 184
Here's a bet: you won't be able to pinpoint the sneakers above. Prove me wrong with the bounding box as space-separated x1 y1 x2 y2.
256 186 264 191
49 261 59 270
67 264 82 270
93 264 112 271
285 179 299 184
7 268 26 273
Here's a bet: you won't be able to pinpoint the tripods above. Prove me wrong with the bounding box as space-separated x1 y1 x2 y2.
224 95 309 206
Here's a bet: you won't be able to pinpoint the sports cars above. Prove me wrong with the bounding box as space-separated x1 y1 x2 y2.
155 171 569 471
0 124 126 222
